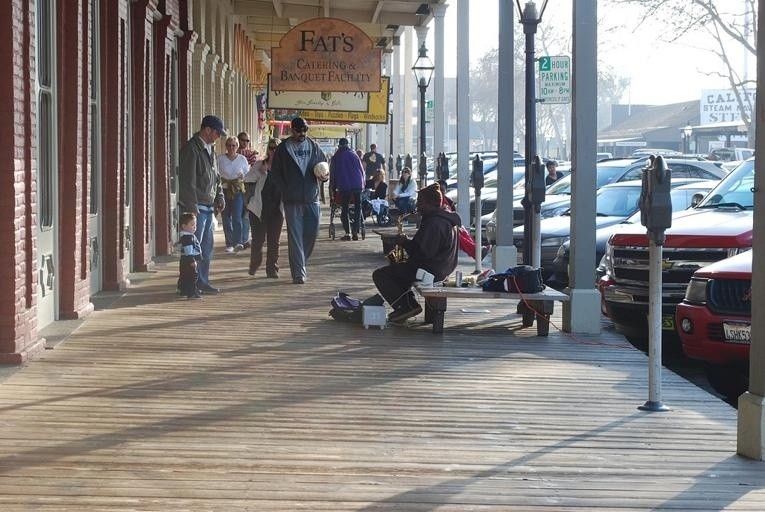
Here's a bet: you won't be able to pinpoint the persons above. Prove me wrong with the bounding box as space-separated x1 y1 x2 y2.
546 161 564 186
244 137 284 279
391 167 418 216
273 117 329 285
331 137 388 240
179 115 228 296
216 132 257 251
372 180 492 323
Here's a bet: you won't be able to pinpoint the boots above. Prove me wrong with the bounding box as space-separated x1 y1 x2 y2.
389 291 422 321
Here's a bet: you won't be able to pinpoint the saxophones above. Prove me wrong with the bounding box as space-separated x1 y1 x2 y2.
384 211 417 264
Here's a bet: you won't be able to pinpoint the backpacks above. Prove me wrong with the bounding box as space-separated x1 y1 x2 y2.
482 265 546 292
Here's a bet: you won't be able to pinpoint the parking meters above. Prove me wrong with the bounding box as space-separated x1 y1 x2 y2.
635 153 677 413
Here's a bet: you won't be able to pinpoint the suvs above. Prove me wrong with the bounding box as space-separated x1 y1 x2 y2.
676 250 756 400
602 154 757 338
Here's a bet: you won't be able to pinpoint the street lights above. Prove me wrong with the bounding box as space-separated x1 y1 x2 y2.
679 128 687 154
347 128 354 151
352 122 361 153
511 1 554 316
682 120 693 155
412 40 437 191
386 82 394 161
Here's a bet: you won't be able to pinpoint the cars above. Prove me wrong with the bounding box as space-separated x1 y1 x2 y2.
387 145 758 290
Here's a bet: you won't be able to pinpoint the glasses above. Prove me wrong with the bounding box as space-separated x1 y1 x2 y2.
293 127 307 133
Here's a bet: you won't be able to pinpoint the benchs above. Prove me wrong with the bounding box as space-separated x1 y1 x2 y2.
412 278 572 337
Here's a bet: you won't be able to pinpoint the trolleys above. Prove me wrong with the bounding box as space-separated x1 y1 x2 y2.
328 188 366 242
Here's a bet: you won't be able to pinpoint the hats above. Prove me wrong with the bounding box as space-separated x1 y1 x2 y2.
415 181 443 205
291 118 309 128
339 138 348 144
202 115 227 137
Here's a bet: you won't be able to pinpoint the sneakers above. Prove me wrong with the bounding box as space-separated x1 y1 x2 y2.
176 284 220 300
249 264 256 274
480 245 492 261
294 275 306 284
352 235 358 240
266 271 279 278
224 238 252 252
340 236 350 240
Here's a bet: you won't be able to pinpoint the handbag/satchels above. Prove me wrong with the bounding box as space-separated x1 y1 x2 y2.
243 180 256 204
